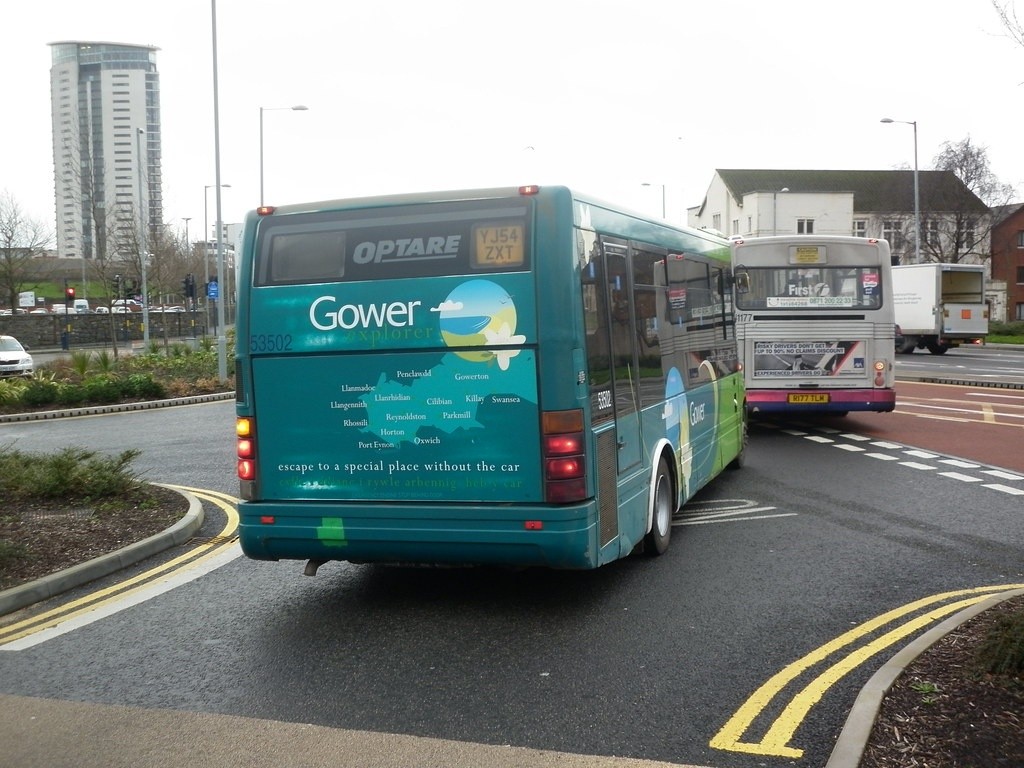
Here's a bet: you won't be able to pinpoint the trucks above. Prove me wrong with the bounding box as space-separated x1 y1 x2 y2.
840 262 990 355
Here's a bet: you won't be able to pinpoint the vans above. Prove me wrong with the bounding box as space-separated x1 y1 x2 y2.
74 299 89 312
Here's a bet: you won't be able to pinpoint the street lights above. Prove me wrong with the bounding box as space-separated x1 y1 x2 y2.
181 217 194 269
204 184 232 336
774 187 790 236
880 117 920 263
642 183 666 220
136 127 144 351
261 106 309 208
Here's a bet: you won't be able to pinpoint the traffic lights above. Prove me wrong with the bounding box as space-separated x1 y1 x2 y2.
110 274 121 300
67 287 74 301
182 278 190 298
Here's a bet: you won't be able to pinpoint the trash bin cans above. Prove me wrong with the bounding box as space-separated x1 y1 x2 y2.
61 332 69 350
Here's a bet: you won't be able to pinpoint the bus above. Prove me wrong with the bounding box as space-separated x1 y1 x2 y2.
728 234 898 424
232 185 748 577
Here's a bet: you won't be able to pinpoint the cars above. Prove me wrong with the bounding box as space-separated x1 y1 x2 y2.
0 299 198 317
0 335 34 381
